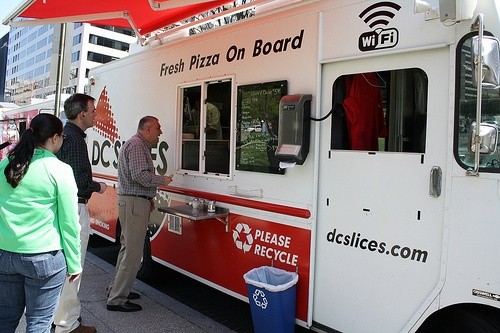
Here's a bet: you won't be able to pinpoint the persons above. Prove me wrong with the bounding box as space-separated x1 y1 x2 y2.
189 91 222 140
0 113 83 333
106 116 172 312
53 93 107 333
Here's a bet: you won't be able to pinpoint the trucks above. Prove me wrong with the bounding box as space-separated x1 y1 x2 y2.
0 0 500 333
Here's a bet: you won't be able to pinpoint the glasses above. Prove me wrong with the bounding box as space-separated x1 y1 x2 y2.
56 134 66 139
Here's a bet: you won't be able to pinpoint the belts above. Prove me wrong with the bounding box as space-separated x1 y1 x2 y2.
78 198 88 204
125 194 152 201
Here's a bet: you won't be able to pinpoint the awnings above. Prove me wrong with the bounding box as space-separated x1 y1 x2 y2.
2 0 235 47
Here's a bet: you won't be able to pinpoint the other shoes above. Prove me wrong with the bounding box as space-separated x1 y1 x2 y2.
107 291 140 300
69 323 97 333
105 300 143 312
51 315 82 328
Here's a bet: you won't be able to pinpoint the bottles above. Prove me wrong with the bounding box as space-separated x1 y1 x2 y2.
207 201 215 212
193 198 199 208
199 199 205 210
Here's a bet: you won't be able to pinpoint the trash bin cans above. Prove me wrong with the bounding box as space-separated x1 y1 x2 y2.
243 266 299 333
